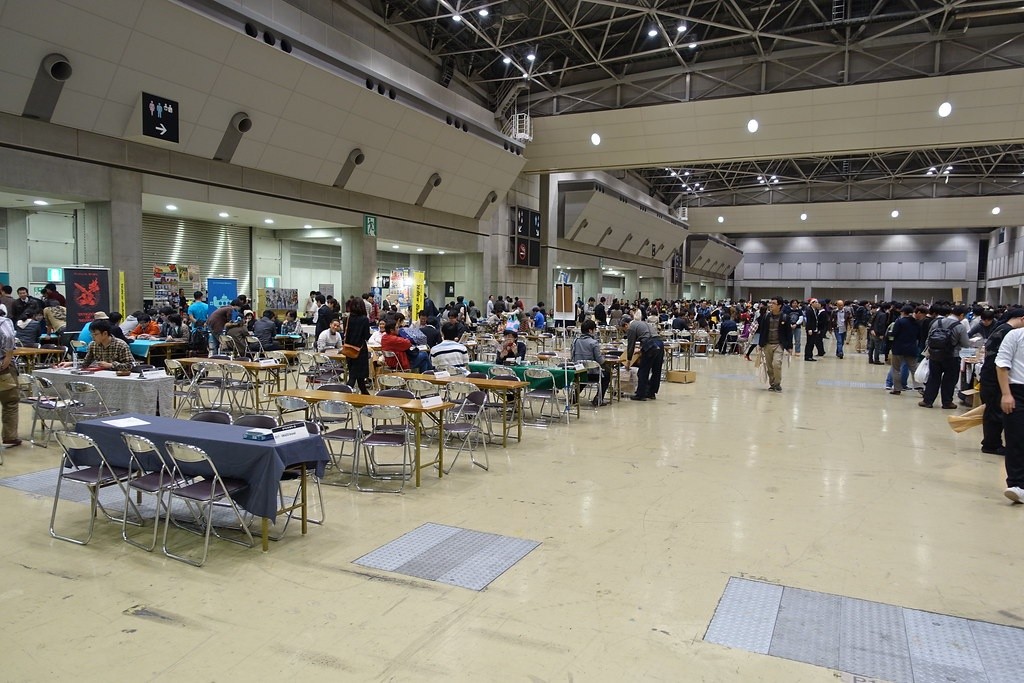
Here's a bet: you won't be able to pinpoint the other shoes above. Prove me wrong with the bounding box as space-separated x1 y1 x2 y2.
918 389 925 396
631 394 656 401
805 357 817 361
890 390 900 395
1004 486 1024 503
982 445 1006 454
942 403 958 409
918 401 934 408
886 387 893 390
869 361 885 365
745 354 751 360
592 396 607 406
768 383 782 391
3 439 22 445
902 387 913 390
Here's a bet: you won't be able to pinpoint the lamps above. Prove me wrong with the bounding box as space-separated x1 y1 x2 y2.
479 2 490 16
676 19 686 32
502 51 512 62
527 49 536 60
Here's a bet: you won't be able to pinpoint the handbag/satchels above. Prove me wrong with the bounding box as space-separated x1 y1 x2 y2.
341 344 361 358
188 327 208 350
640 339 660 360
464 304 471 324
913 356 930 384
406 346 420 357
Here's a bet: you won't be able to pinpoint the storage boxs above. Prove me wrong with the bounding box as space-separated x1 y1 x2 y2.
962 391 982 410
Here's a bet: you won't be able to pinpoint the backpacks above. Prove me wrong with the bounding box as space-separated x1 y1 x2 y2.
927 318 962 360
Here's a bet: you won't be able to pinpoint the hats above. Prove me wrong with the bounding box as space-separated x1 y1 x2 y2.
806 297 817 304
244 309 254 317
94 311 109 319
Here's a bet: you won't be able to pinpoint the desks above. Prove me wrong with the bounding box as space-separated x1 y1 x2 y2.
525 334 549 352
13 346 65 375
469 360 588 422
248 330 303 351
604 333 720 402
275 349 374 388
268 389 455 488
30 367 176 419
65 413 331 553
374 371 531 448
128 338 191 364
175 356 285 414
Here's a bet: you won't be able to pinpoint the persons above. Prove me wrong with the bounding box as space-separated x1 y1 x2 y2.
571 297 1023 502
0 282 554 444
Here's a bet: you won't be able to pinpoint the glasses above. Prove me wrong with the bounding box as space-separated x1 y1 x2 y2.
89 331 101 337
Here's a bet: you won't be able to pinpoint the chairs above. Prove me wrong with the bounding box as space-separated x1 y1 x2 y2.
721 330 740 356
14 326 710 570
